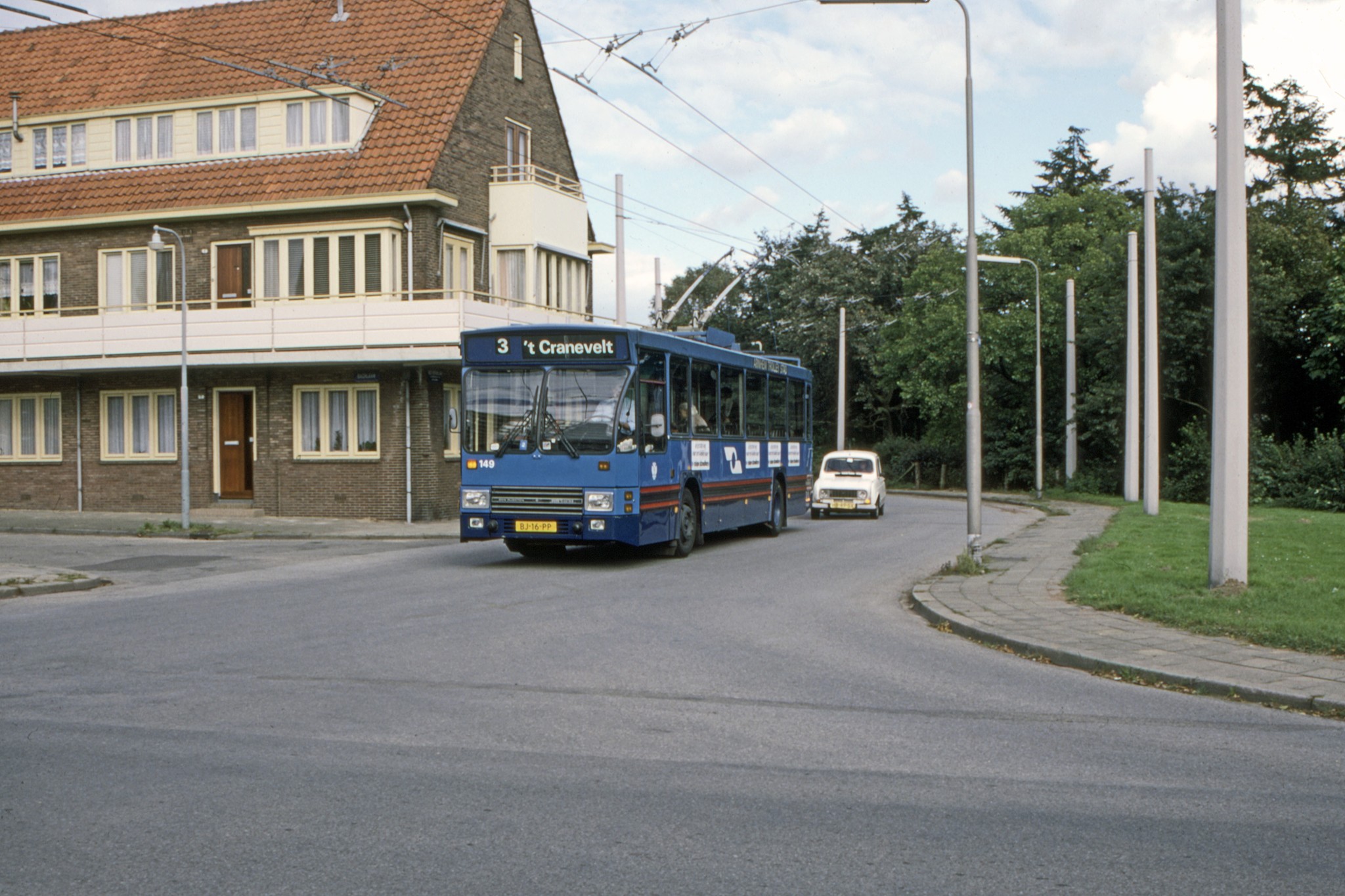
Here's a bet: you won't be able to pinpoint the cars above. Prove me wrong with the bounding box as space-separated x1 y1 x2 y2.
810 449 887 520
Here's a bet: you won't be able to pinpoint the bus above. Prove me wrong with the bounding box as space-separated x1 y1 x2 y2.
458 322 814 563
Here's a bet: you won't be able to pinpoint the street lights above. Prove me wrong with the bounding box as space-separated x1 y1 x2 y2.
978 253 1044 497
148 224 190 529
816 0 984 565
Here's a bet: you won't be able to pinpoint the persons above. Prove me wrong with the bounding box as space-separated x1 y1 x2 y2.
859 461 869 472
678 403 710 433
498 403 530 441
587 378 636 436
